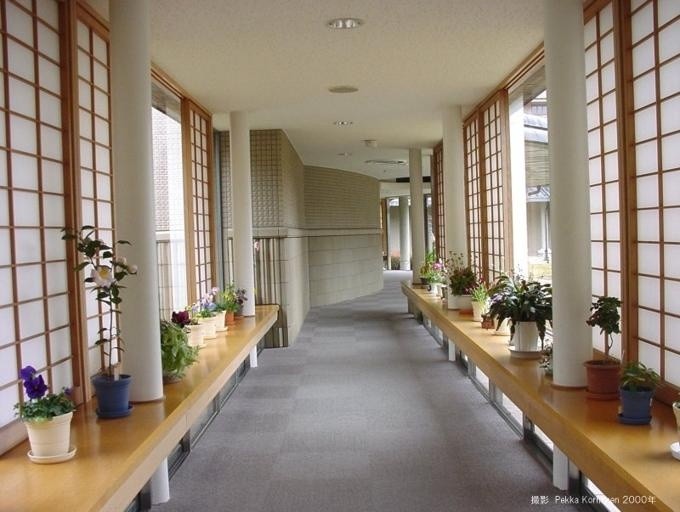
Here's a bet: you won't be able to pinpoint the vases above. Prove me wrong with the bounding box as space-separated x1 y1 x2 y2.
89 372 133 423
18 408 78 466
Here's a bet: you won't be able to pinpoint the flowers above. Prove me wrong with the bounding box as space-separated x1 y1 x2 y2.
155 281 250 385
53 217 144 382
12 364 83 426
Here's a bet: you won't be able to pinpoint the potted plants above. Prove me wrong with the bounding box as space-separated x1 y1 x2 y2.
417 247 680 462
